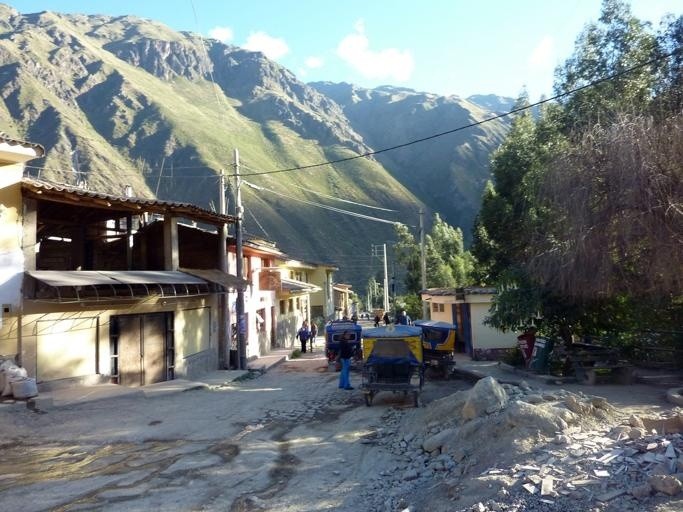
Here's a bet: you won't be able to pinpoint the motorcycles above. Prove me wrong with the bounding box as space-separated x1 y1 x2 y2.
324 320 457 407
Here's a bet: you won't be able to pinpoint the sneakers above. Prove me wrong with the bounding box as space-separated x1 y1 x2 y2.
339 386 354 390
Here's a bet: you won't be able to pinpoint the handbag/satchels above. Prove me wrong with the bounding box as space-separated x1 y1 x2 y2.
334 362 342 372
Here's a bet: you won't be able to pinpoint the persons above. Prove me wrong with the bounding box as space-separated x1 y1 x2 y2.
382 312 389 326
296 321 311 353
336 330 354 390
398 310 411 326
374 314 380 328
309 324 318 353
